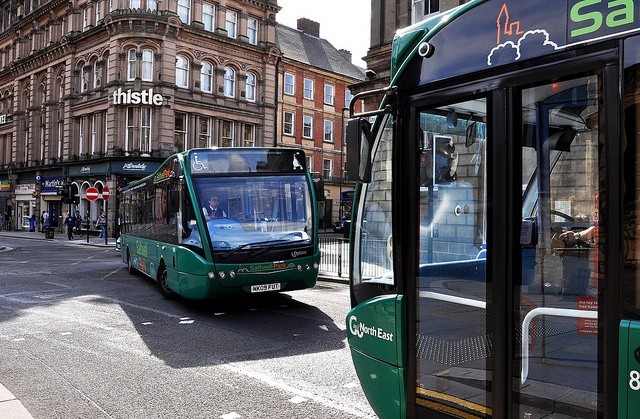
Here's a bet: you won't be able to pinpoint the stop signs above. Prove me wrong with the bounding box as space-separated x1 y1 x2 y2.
84 188 100 202
102 186 111 201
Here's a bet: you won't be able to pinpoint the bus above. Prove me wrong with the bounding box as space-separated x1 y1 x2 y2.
119 146 321 301
345 0 640 418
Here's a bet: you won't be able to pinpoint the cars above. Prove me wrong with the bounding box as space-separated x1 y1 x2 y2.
334 216 346 232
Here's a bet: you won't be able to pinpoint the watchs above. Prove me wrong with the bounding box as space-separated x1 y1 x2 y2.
574 232 580 244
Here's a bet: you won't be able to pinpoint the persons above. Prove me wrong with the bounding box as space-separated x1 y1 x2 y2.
75 210 82 237
40 211 46 232
202 195 228 220
64 214 75 240
561 225 599 248
95 211 106 240
43 210 49 226
3 211 10 231
0 213 4 231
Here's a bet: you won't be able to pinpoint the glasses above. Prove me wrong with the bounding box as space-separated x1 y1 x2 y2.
212 199 220 202
